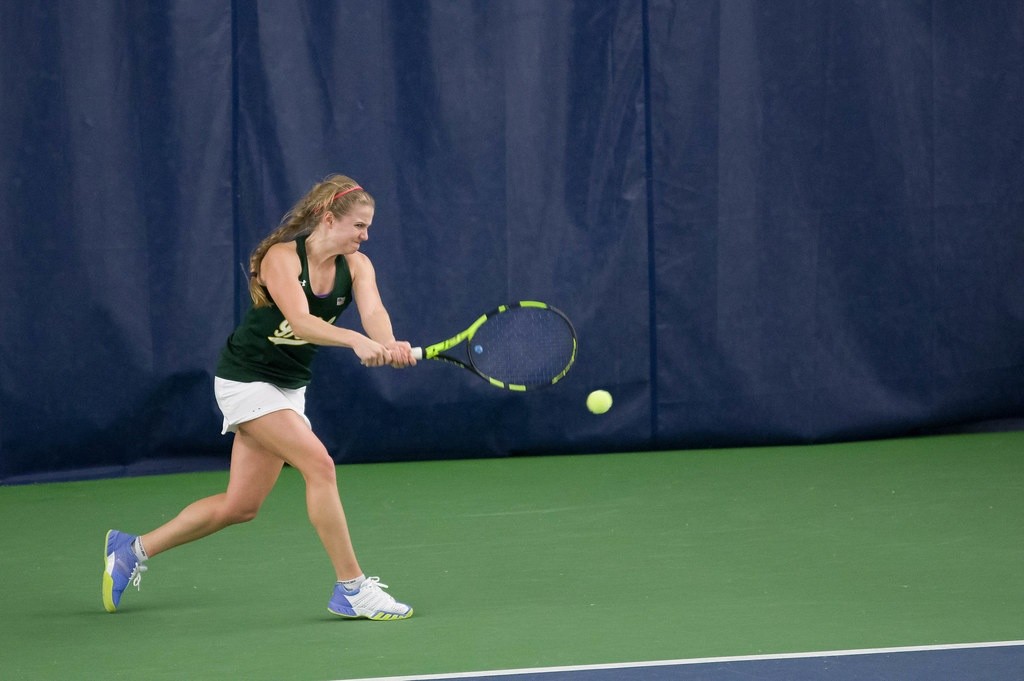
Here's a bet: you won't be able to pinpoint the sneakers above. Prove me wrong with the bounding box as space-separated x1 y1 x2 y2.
103 530 147 612
327 578 413 620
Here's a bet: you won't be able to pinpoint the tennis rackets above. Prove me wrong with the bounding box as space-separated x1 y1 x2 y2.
360 300 579 393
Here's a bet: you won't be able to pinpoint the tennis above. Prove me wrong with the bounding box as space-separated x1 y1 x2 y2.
586 389 614 415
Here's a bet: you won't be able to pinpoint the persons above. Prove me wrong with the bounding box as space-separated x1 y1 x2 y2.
103 173 418 621
